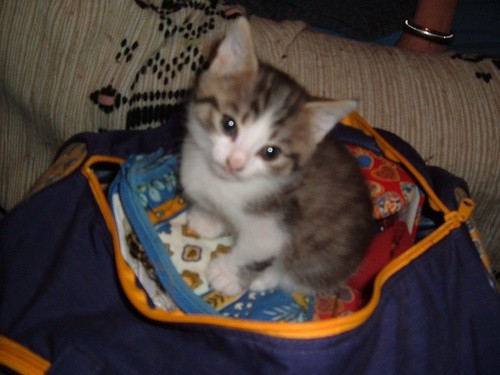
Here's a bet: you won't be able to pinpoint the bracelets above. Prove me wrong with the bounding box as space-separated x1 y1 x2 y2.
400 18 456 47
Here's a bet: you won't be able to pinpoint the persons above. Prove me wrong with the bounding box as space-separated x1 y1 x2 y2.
302 1 467 58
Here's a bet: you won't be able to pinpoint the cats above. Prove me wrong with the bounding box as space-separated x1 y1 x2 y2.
179 16 374 297
